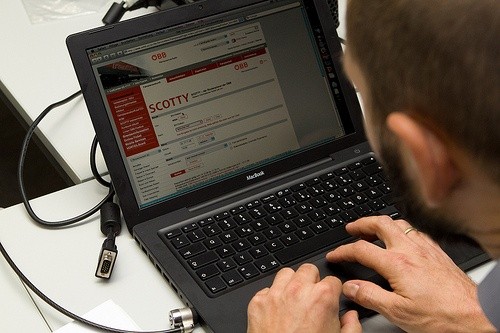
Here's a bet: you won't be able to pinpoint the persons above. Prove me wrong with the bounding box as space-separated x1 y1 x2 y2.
246 1 499 332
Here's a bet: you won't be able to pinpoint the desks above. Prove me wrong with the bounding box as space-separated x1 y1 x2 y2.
0 0 500 333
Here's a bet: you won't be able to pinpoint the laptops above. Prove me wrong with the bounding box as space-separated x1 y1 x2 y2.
66 0 493 333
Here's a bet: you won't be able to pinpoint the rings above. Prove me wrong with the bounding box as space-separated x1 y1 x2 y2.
405 226 419 233
